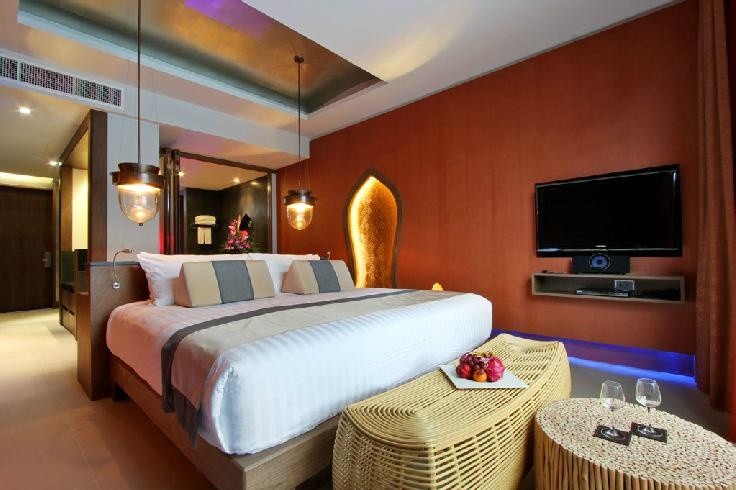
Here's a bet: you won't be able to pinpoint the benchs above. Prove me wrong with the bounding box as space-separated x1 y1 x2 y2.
333 335 573 490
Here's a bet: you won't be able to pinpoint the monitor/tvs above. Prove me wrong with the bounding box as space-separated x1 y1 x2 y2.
532 161 684 258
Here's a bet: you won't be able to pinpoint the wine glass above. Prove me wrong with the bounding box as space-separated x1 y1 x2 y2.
600 380 626 440
635 378 664 437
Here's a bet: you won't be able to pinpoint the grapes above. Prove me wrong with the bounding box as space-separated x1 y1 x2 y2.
460 353 487 374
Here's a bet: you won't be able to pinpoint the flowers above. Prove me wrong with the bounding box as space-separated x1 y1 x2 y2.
224 220 256 254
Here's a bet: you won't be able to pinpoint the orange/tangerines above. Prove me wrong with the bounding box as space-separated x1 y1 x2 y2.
472 369 487 382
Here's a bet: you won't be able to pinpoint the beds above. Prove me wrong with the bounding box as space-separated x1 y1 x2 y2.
84 262 492 490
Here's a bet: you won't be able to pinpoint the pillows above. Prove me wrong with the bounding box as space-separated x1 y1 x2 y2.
281 259 354 293
137 252 319 304
174 258 273 306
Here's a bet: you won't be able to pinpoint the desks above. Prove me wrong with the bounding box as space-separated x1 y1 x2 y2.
533 398 736 490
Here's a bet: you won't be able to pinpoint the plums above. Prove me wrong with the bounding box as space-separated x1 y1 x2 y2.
457 363 471 378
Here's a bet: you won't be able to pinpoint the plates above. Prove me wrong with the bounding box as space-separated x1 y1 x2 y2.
439 364 528 389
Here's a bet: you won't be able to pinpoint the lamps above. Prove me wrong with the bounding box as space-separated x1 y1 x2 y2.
113 0 165 226
285 55 317 231
